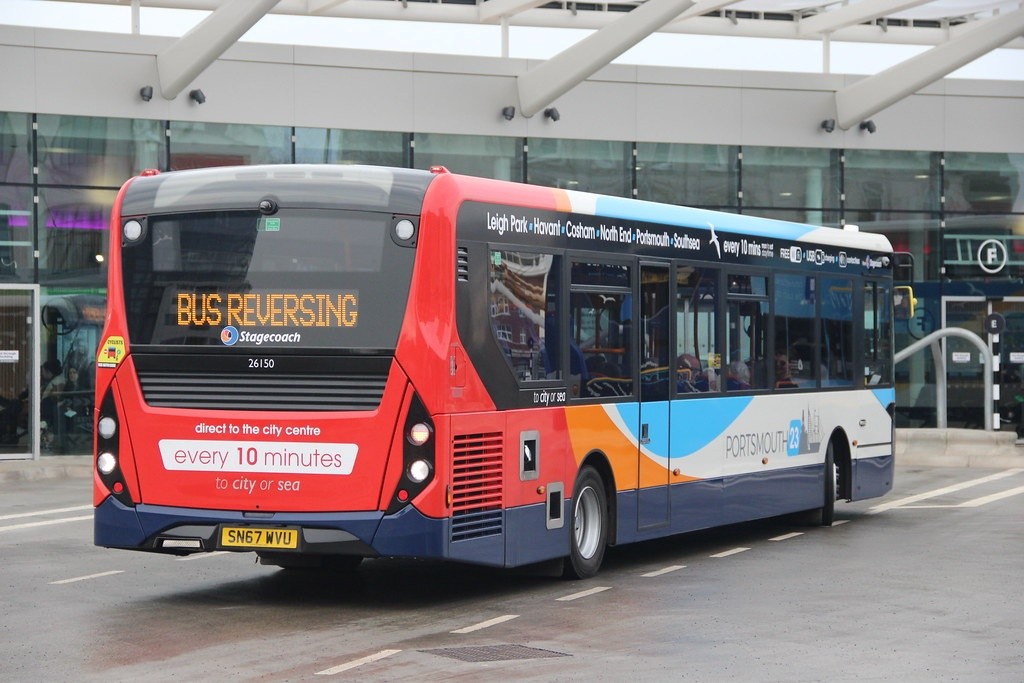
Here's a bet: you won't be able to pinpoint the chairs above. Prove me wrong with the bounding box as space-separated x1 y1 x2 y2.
542 337 830 398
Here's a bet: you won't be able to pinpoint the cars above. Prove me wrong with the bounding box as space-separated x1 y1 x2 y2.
947 311 985 334
788 341 825 376
1004 311 1024 330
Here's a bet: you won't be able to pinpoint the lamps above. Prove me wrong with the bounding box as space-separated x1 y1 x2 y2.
860 119 878 134
501 106 520 122
545 108 562 123
190 89 204 104
819 118 837 136
140 87 154 101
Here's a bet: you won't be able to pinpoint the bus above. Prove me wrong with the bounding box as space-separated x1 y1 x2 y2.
894 369 1022 406
94 164 918 578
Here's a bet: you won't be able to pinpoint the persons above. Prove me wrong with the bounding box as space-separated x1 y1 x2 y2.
678 350 793 391
